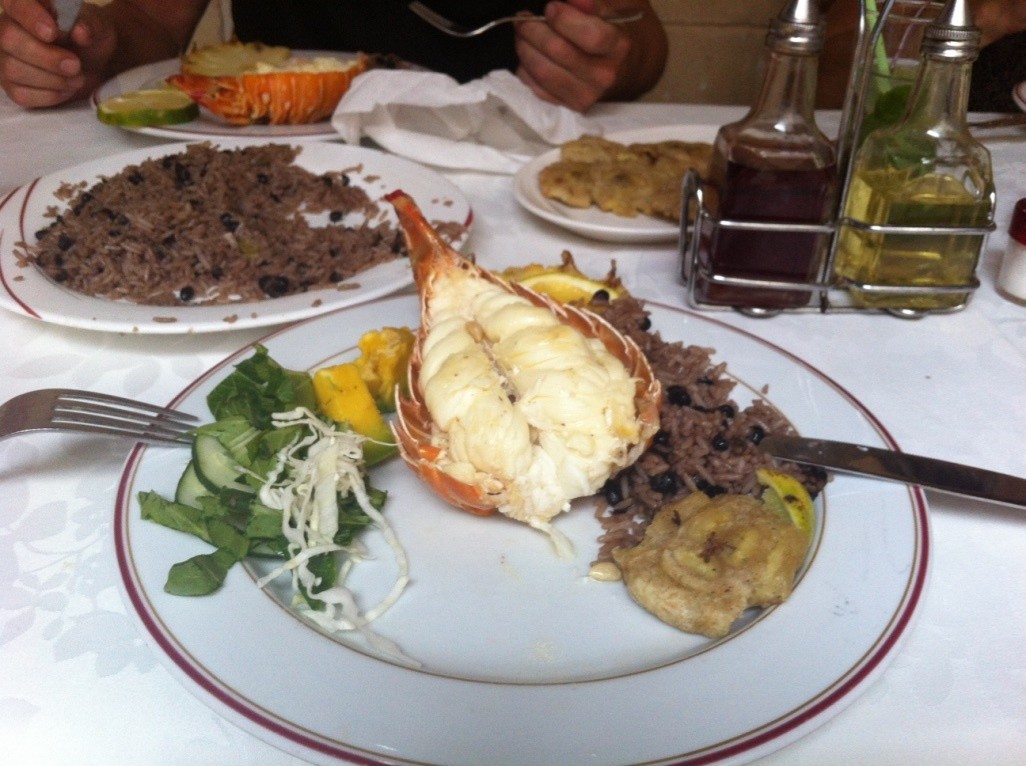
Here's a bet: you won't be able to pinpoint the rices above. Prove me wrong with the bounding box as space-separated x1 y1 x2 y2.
566 282 833 588
2 134 457 301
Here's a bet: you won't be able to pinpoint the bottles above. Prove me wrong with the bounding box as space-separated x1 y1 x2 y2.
693 0 841 306
832 0 994 318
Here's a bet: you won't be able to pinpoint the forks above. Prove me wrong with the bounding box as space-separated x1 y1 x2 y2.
413 1 643 40
0 390 202 450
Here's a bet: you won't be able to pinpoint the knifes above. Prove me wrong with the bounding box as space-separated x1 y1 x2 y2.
761 434 1026 514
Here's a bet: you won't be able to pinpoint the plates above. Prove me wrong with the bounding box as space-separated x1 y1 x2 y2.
117 285 935 766
514 122 724 245
2 138 474 333
93 47 437 140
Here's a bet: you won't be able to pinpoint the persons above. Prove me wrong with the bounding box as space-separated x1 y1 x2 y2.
0 0 671 116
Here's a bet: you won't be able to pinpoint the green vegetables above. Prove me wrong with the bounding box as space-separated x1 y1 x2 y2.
134 342 387 638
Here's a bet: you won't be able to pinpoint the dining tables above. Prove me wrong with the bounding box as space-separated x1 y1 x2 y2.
0 95 1024 766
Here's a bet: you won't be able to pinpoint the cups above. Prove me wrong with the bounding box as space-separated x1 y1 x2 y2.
837 1 945 183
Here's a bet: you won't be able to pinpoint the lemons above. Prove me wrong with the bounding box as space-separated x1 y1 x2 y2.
96 89 195 128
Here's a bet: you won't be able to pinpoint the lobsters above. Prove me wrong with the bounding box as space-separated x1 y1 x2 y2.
164 48 401 125
378 187 661 543
